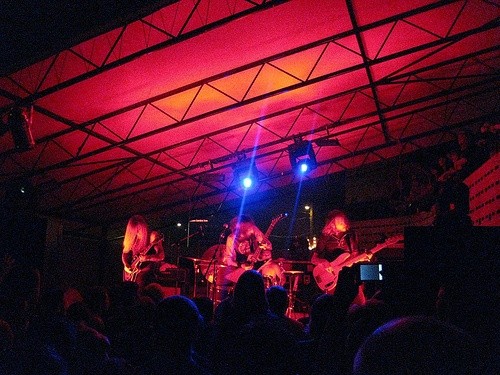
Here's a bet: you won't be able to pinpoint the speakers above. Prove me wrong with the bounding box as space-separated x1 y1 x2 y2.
177 256 220 300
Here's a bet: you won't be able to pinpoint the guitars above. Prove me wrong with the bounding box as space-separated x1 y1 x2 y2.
313 233 403 291
225 214 288 283
123 236 163 282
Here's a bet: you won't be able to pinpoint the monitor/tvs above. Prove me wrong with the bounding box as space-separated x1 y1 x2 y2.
356 263 384 281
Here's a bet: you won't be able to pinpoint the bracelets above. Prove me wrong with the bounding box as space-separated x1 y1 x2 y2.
148 255 150 261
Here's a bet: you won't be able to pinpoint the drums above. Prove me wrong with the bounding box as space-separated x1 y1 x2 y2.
210 286 233 302
286 289 296 309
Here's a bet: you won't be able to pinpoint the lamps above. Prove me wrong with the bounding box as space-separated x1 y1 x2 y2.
232 159 260 193
7 98 35 152
288 134 318 176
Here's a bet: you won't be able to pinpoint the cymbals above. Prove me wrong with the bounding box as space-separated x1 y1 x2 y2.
184 256 211 262
198 264 226 268
284 270 304 275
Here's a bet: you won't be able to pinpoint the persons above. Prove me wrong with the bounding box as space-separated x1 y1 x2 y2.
0 248 500 375
311 210 373 306
226 214 272 285
122 215 165 287
263 262 286 287
